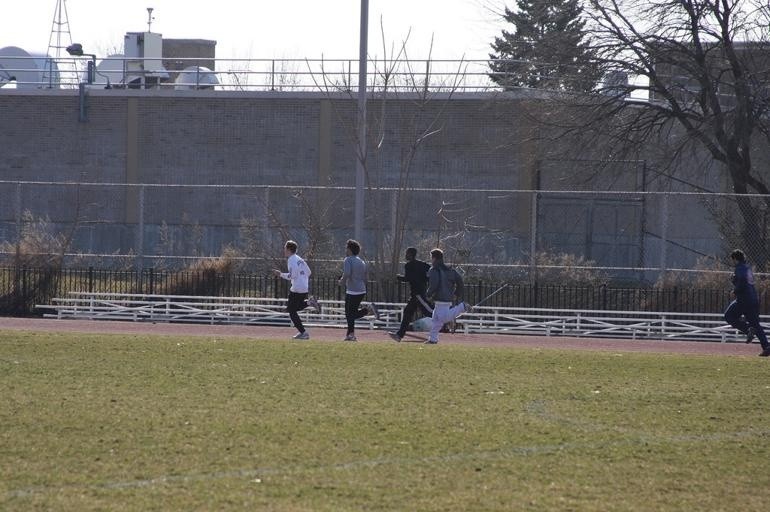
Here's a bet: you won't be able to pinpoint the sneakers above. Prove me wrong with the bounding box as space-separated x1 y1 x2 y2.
462 301 474 313
309 296 321 313
423 340 437 344
388 331 401 342
744 326 758 344
344 332 357 341
368 303 380 320
293 331 310 339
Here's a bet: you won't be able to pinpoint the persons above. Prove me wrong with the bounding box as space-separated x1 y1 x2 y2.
271 240 321 339
423 248 475 342
338 238 380 341
723 249 770 357
387 247 457 344
